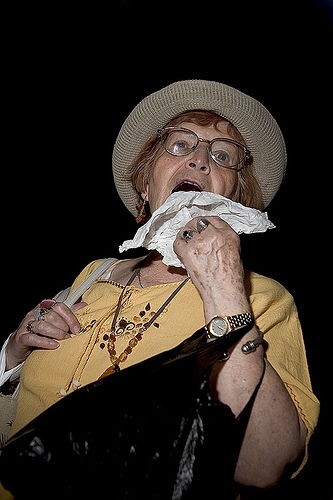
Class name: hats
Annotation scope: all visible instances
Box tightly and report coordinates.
[111,78,288,221]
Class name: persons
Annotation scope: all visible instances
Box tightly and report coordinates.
[0,78,320,500]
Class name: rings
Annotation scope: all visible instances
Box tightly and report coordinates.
[196,218,211,234]
[38,307,52,321]
[182,228,199,242]
[26,320,39,334]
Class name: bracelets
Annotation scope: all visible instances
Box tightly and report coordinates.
[217,338,269,362]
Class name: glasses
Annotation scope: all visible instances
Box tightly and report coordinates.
[153,125,252,169]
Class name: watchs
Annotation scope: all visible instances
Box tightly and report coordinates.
[204,311,255,340]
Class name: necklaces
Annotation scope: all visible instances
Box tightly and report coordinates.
[97,255,192,381]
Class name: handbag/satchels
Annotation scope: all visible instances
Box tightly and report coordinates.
[1,258,122,444]
[0,320,266,500]
